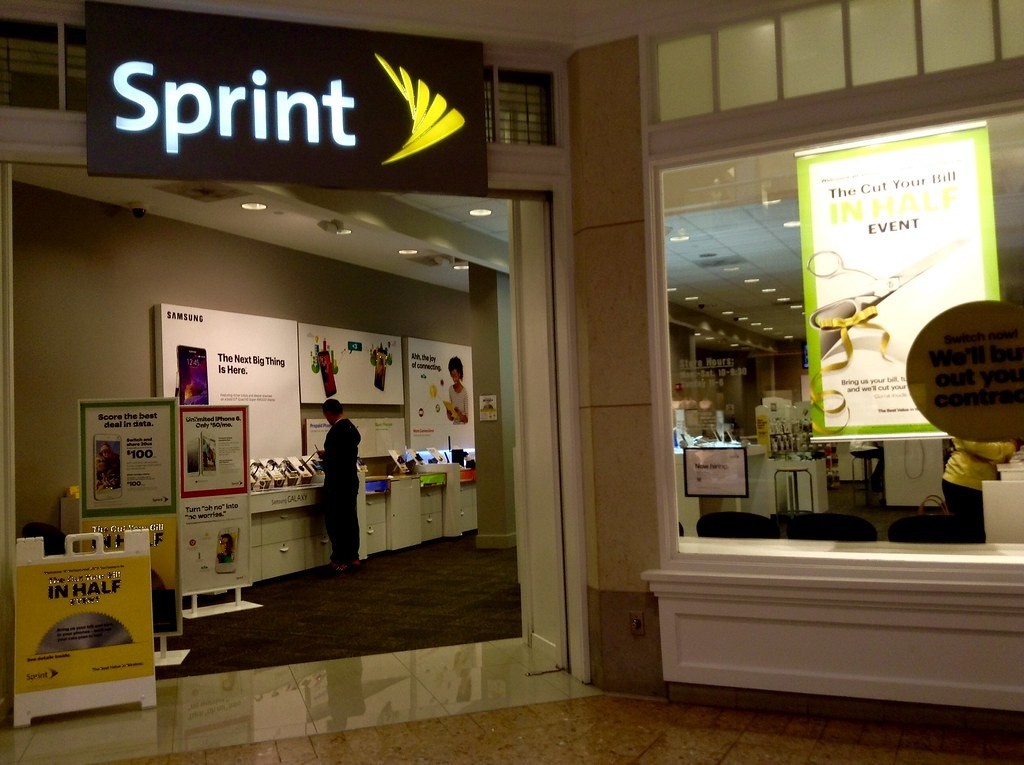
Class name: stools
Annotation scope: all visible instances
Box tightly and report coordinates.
[774,466,814,519]
[851,447,884,508]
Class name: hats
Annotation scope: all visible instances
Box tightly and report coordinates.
[99,445,111,453]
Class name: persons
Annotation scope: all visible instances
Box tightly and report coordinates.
[941,434,1022,530]
[96,442,121,494]
[445,356,469,424]
[216,534,238,566]
[316,398,362,572]
[849,441,886,492]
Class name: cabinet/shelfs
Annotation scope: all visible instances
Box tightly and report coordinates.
[305,503,333,570]
[459,480,478,532]
[262,506,305,581]
[420,485,443,542]
[365,494,387,555]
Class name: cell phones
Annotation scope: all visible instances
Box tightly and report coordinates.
[317,351,337,399]
[177,345,209,406]
[348,341,362,351]
[374,352,386,391]
[93,435,121,500]
[188,431,217,477]
[215,527,239,575]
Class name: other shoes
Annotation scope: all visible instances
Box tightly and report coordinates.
[325,562,339,572]
[333,560,361,577]
